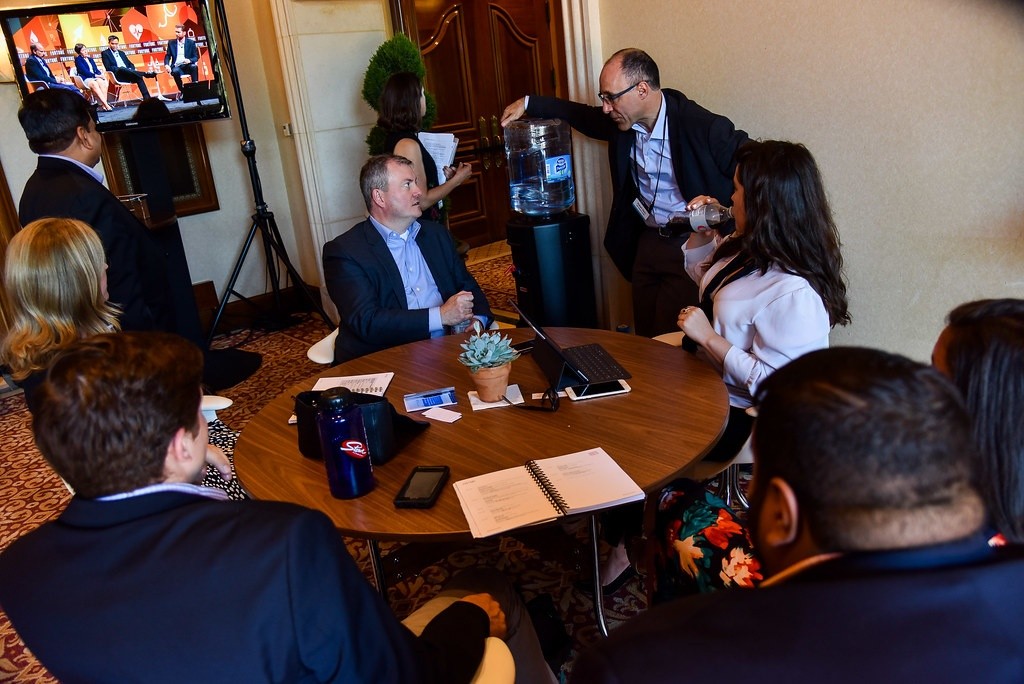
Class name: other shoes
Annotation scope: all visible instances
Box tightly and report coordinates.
[102,106,107,111]
[603,563,637,595]
[108,107,112,111]
[179,91,183,100]
[145,72,157,77]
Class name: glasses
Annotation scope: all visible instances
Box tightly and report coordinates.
[598,80,650,104]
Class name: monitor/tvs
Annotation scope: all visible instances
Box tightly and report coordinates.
[0,0,232,134]
[182,80,218,103]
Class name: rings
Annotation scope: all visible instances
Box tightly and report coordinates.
[683,307,690,314]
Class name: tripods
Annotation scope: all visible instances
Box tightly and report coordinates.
[207,0,336,347]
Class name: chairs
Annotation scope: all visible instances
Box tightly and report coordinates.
[167,62,198,101]
[69,67,115,109]
[106,71,144,108]
[24,74,50,92]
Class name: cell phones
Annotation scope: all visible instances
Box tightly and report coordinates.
[393,466,451,509]
[511,338,535,354]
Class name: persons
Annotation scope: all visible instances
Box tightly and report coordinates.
[16,88,202,352]
[568,346,1024,684]
[74,43,113,112]
[101,36,157,99]
[369,71,474,225]
[164,24,200,100]
[3,216,249,503]
[497,47,754,340]
[25,42,98,109]
[323,155,495,368]
[1,334,557,684]
[598,140,853,591]
[929,298,1024,543]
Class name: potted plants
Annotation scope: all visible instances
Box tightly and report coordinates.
[455,321,522,402]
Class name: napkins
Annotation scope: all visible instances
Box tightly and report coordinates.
[467,384,525,412]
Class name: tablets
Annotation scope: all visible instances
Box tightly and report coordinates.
[506,298,587,385]
[565,379,631,401]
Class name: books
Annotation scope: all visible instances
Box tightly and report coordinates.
[453,446,647,540]
[288,371,395,425]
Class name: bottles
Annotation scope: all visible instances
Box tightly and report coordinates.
[658,204,732,238]
[504,119,575,221]
[453,320,472,335]
[317,387,376,499]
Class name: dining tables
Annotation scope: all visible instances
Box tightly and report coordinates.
[232,327,732,639]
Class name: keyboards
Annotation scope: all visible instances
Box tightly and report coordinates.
[564,343,632,383]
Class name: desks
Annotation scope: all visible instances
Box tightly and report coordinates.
[146,70,172,101]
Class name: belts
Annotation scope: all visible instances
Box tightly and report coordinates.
[651,227,688,238]
